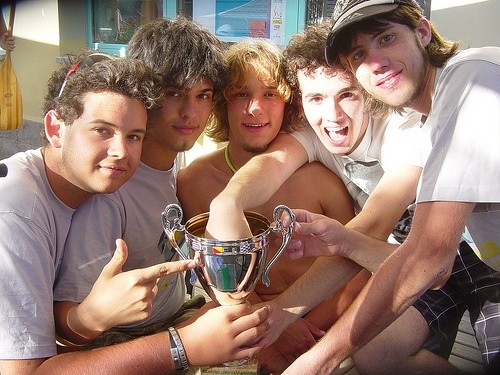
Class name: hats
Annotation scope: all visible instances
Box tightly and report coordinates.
[324,0,420,65]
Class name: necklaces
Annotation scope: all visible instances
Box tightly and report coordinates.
[224,143,238,174]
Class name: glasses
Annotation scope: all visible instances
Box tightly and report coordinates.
[58,53,123,99]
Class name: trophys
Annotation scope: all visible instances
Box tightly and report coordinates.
[161,203,296,375]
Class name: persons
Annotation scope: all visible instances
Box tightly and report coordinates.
[204,18,500,375]
[271,0,500,375]
[53,17,231,375]
[0,48,274,375]
[176,39,372,375]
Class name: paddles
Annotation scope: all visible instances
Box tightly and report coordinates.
[0,0,23,130]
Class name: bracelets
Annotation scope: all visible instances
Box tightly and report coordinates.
[167,326,189,375]
